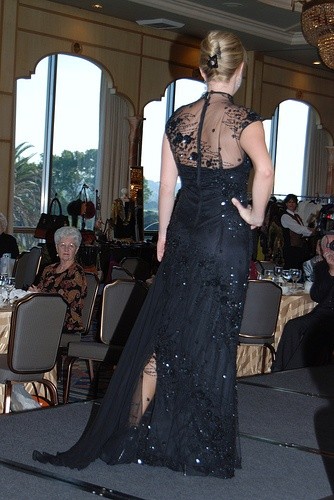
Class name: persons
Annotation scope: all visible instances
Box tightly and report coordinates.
[281,194,314,269]
[146,255,160,285]
[0,212,19,259]
[248,200,270,261]
[149,234,158,247]
[28,226,87,381]
[271,230,334,372]
[261,221,289,258]
[33,30,273,478]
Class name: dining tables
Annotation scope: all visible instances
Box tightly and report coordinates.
[82,240,150,269]
[237,283,319,379]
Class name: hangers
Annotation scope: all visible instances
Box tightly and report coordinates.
[301,196,320,207]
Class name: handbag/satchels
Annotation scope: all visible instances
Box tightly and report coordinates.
[34,198,70,239]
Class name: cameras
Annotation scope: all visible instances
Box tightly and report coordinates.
[329,240,334,250]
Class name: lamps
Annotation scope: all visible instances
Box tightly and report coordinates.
[291,0,334,70]
[136,11,185,29]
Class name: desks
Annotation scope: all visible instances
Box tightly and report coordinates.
[0,291,52,352]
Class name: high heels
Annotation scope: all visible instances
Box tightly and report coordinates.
[116,425,138,462]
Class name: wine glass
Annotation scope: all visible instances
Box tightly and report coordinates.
[264,266,302,290]
[0,273,16,306]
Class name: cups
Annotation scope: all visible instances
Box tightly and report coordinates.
[274,279,287,287]
[281,287,288,294]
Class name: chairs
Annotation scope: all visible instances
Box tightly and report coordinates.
[13,247,42,288]
[63,255,141,403]
[238,280,282,374]
[0,294,69,412]
